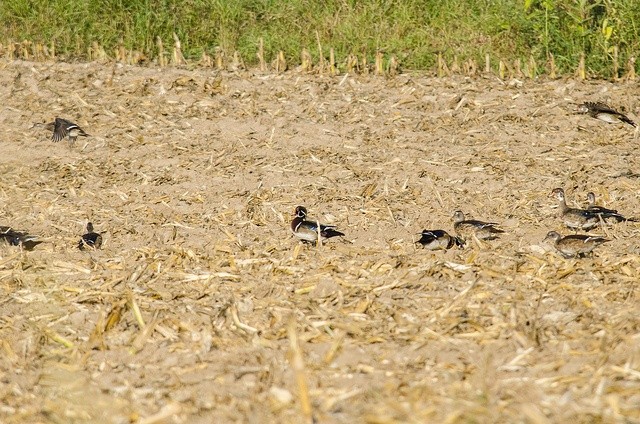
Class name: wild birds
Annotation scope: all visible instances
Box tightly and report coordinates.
[1,221,55,252]
[413,209,505,257]
[45,116,92,147]
[77,221,104,253]
[569,97,640,131]
[289,204,345,248]
[540,183,636,260]
